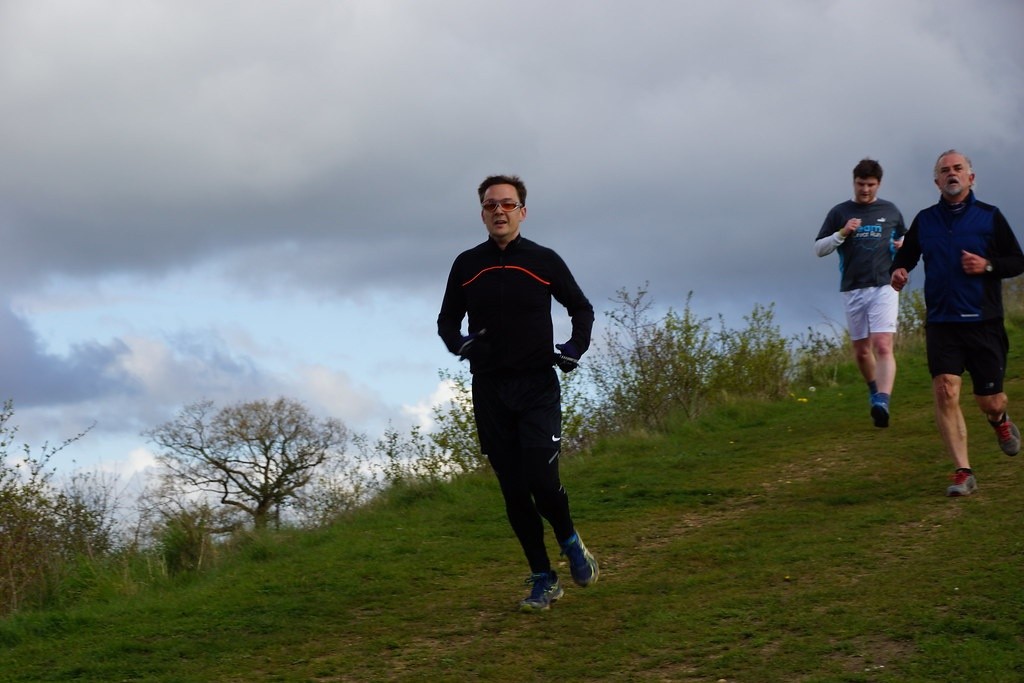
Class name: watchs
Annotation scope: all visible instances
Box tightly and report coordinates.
[985,260,993,272]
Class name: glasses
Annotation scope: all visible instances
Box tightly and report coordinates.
[481,197,523,212]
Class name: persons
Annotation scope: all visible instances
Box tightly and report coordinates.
[889,149,1024,495]
[815,160,907,428]
[438,176,595,611]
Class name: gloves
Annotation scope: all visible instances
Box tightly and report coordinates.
[459,328,486,363]
[556,342,581,373]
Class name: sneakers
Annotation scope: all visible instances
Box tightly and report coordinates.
[870,393,890,428]
[518,570,564,613]
[560,529,600,588]
[986,414,1021,457]
[946,470,977,497]
